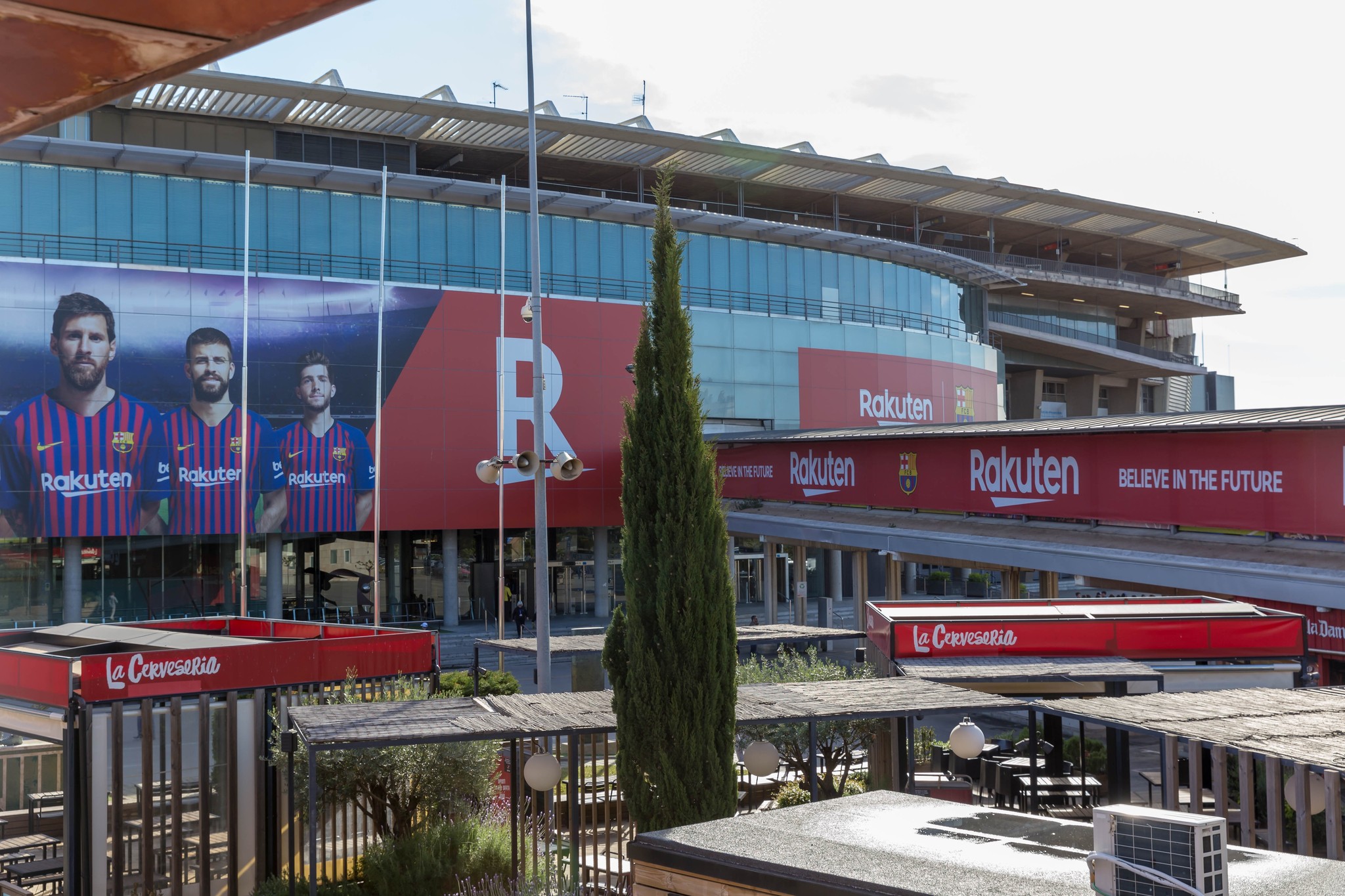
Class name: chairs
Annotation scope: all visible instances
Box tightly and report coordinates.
[579,849,626,896]
[141,784,173,818]
[540,838,570,889]
[927,742,1075,812]
[180,780,214,812]
[36,793,64,834]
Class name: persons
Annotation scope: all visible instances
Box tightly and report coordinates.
[407,594,436,619]
[144,328,287,534]
[1075,591,1155,598]
[0,293,171,537]
[577,566,586,582]
[531,612,536,624]
[748,615,759,659]
[512,601,529,639]
[263,351,375,532]
[749,567,756,598]
[504,581,512,622]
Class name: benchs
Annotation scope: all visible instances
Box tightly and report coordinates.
[0,852,36,873]
[10,872,64,894]
[1018,790,1090,816]
[154,842,198,876]
[753,799,781,814]
[189,858,227,883]
[167,845,228,885]
[1047,802,1104,823]
[0,872,51,891]
[123,826,195,874]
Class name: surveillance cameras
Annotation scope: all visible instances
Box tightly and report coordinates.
[521,305,533,323]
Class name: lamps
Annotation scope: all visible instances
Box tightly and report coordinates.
[949,712,985,758]
[1284,771,1326,815]
[742,722,780,776]
[523,738,562,791]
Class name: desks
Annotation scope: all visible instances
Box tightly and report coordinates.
[0,773,228,896]
[950,743,1000,796]
[1253,828,1292,852]
[562,775,629,828]
[737,756,810,811]
[530,838,571,889]
[1178,787,1216,812]
[816,749,869,775]
[737,791,746,801]
[1139,771,1161,808]
[998,757,1047,812]
[1018,775,1103,815]
[1187,807,1260,847]
[562,853,632,896]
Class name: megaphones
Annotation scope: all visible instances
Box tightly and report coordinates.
[550,452,584,481]
[475,455,502,484]
[512,450,540,477]
